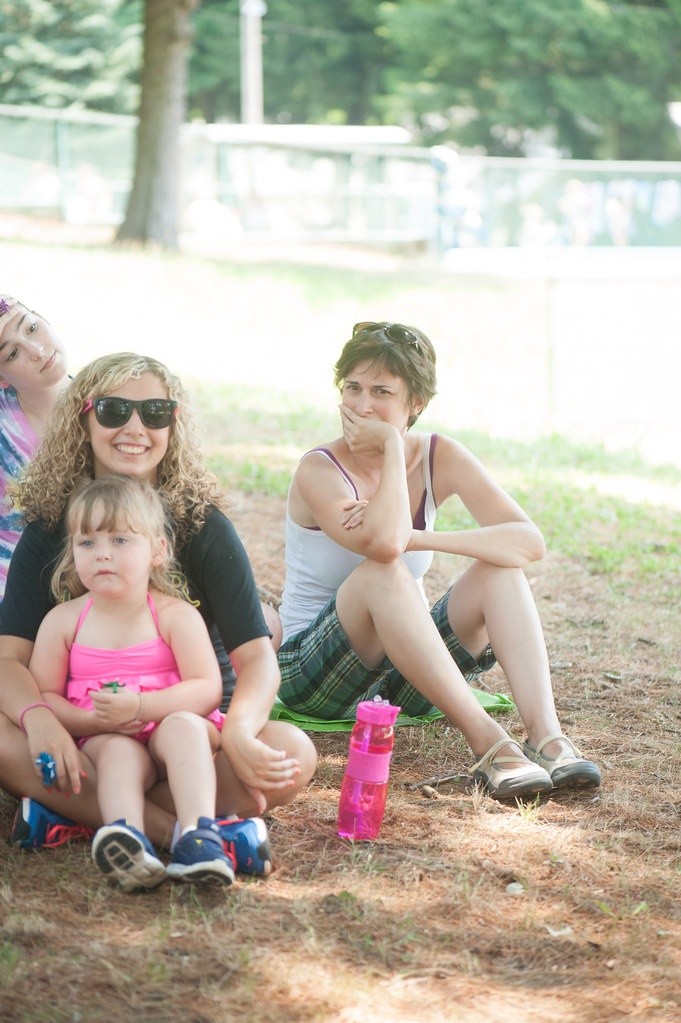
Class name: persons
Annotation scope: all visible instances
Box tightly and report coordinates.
[277,322,601,799]
[0,291,318,892]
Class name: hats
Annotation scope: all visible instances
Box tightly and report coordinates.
[0,296,21,337]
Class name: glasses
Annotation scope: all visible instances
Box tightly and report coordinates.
[81,396,180,429]
[353,321,419,352]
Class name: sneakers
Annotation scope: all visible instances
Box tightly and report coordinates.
[91,818,169,895]
[11,796,92,848]
[166,815,236,885]
[214,814,272,875]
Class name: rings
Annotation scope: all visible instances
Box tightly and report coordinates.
[103,682,127,694]
[37,751,56,786]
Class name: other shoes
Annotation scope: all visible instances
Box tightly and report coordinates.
[468,738,553,799]
[523,733,600,791]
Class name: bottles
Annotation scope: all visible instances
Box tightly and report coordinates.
[337,695,401,841]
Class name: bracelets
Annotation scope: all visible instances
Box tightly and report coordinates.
[18,703,58,734]
[134,693,145,720]
